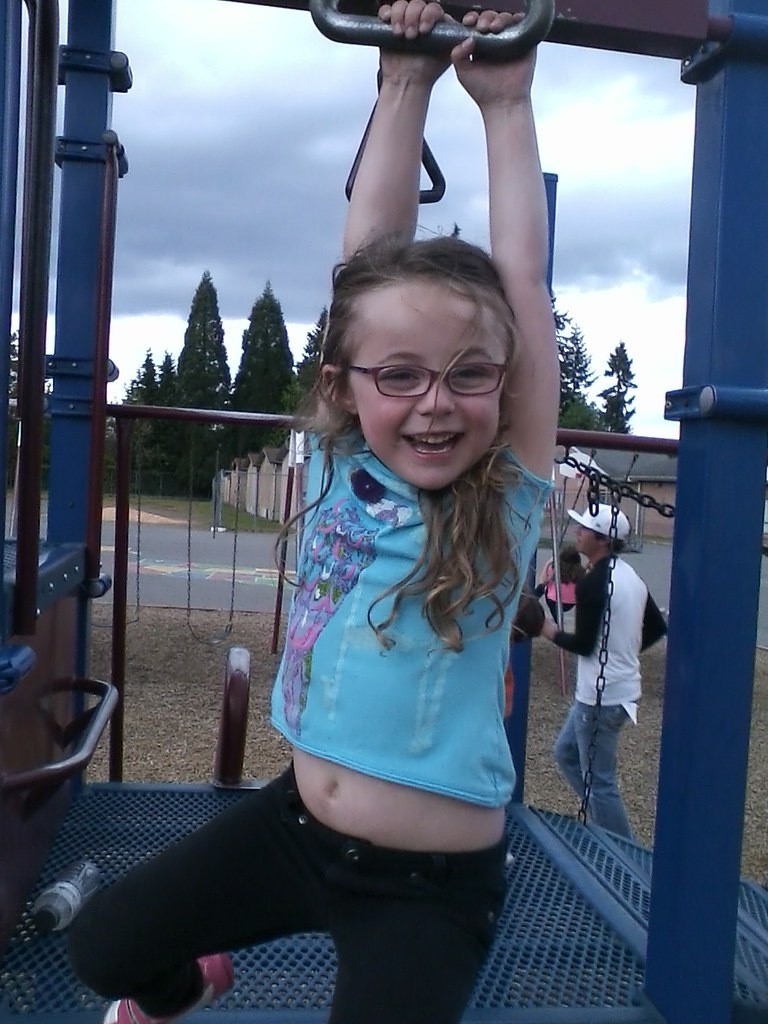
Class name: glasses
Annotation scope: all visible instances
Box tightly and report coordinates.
[347,363,509,396]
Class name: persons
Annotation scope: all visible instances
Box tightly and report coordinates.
[69,0,561,1024]
[544,504,666,838]
[542,545,585,630]
[505,584,546,718]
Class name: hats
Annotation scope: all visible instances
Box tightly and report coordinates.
[567,503,630,541]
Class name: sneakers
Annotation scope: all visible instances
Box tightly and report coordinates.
[103,951,235,1024]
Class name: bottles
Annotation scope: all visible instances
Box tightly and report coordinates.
[34,859,101,932]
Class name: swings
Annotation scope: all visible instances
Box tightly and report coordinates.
[184,420,242,646]
[86,416,146,631]
